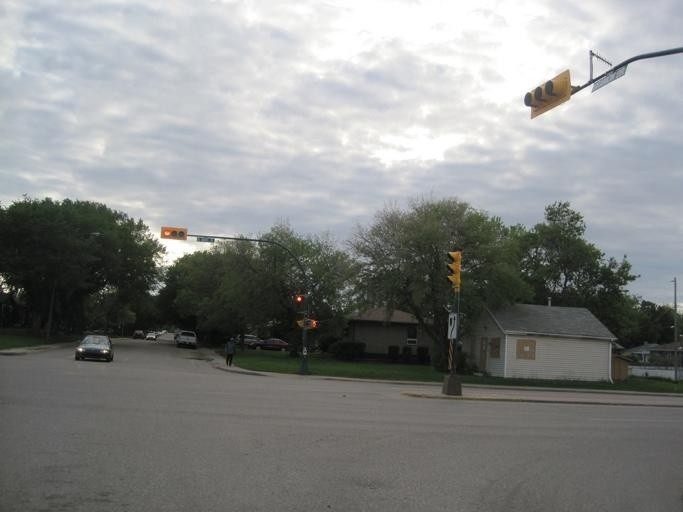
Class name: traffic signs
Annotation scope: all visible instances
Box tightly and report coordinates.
[591,64,628,93]
[197,236,214,243]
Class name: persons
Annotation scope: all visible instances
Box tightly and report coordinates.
[224,337,235,366]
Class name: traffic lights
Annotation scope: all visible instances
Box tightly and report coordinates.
[309,320,319,328]
[524,70,573,119]
[445,251,461,289]
[294,294,306,305]
[161,227,187,240]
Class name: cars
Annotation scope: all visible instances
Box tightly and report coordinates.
[75,335,114,362]
[231,333,292,352]
[133,330,197,349]
